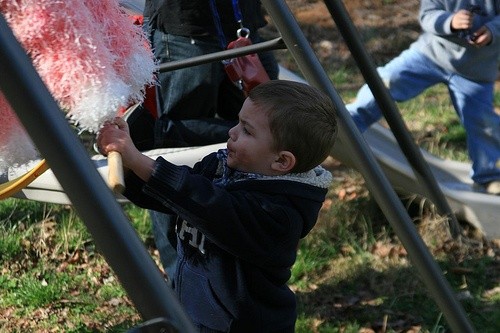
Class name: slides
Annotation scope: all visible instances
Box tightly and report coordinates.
[274,62,500,239]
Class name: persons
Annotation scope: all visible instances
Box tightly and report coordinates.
[141,0,280,294]
[97,79,339,333]
[340,0,500,197]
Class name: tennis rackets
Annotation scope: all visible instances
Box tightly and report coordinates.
[99,20,151,194]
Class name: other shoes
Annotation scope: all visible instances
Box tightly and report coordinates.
[475,177,500,196]
